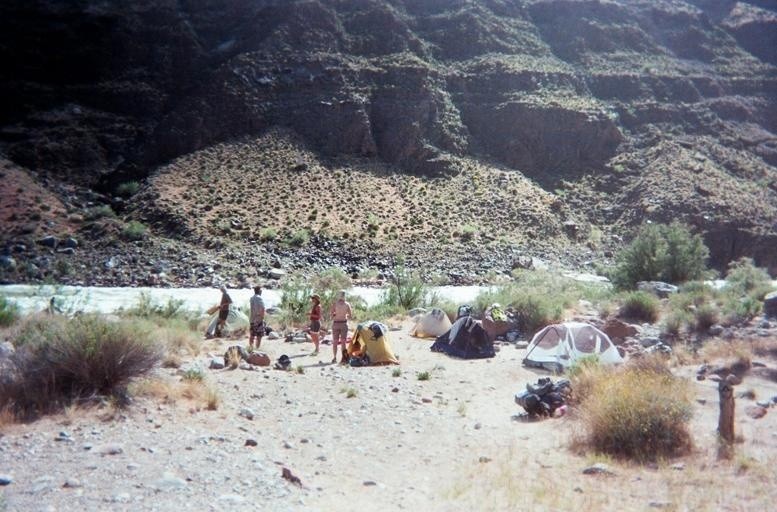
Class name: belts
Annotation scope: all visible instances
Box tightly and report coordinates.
[335,321,346,323]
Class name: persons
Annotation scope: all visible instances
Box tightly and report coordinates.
[329,289,351,364]
[303,293,321,356]
[246,285,266,348]
[213,283,234,337]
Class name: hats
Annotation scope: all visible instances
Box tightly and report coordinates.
[311,295,320,303]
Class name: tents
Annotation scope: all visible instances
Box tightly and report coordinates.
[520,322,625,377]
[342,321,400,368]
[407,306,452,340]
[429,315,496,360]
